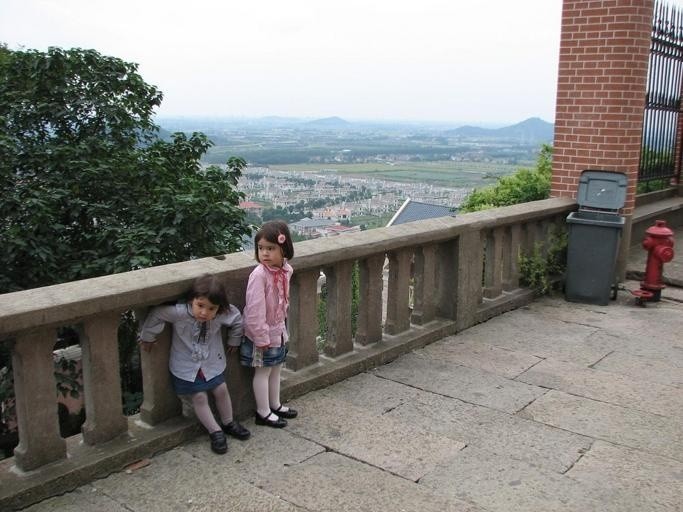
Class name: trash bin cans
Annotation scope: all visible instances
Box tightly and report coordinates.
[564,170,627,305]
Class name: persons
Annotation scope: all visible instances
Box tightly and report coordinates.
[236,220,298,428]
[139,275,250,455]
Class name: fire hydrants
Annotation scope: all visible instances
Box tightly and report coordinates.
[630,221,674,305]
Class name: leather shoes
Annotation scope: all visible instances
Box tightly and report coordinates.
[209,422,250,454]
[255,404,297,428]
